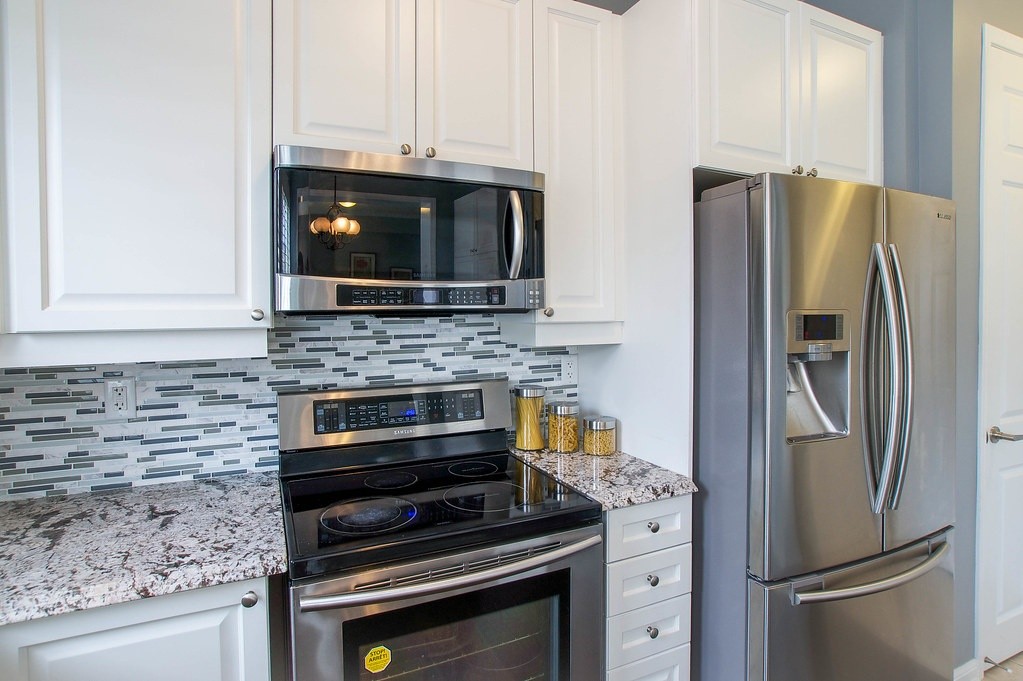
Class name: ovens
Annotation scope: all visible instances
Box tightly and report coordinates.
[288,519,606,680]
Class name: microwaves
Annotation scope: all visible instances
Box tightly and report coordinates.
[273,145,547,312]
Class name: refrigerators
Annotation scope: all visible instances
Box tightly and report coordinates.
[692,173,954,681]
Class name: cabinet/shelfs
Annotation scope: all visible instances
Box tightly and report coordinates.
[0,579,271,679]
[606,492,690,681]
[502,0,623,347]
[3,1,270,373]
[272,1,533,170]
[621,1,885,477]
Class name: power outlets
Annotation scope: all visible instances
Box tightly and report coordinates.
[560,355,578,385]
[104,376,136,419]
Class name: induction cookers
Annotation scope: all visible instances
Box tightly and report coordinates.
[276,380,603,579]
